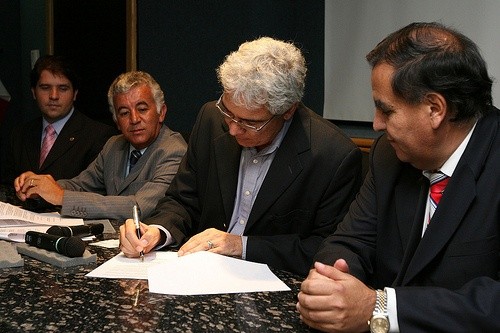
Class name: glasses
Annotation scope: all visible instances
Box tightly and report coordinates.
[216,93,275,132]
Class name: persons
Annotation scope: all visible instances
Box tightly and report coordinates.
[297,22,500,333]
[14,72,189,220]
[119,37,363,277]
[0,56,121,189]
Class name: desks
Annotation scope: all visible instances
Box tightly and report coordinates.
[1,190,329,333]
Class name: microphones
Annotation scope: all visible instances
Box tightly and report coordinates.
[46,222,104,238]
[25,230,86,259]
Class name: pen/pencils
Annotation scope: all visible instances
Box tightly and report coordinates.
[133,201,144,261]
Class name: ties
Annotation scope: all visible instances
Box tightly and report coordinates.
[427,173,451,228]
[39,124,56,169]
[129,150,143,176]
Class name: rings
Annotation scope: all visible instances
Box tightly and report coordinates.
[208,241,213,248]
[29,179,34,186]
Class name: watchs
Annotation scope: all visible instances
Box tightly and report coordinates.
[368,289,390,333]
